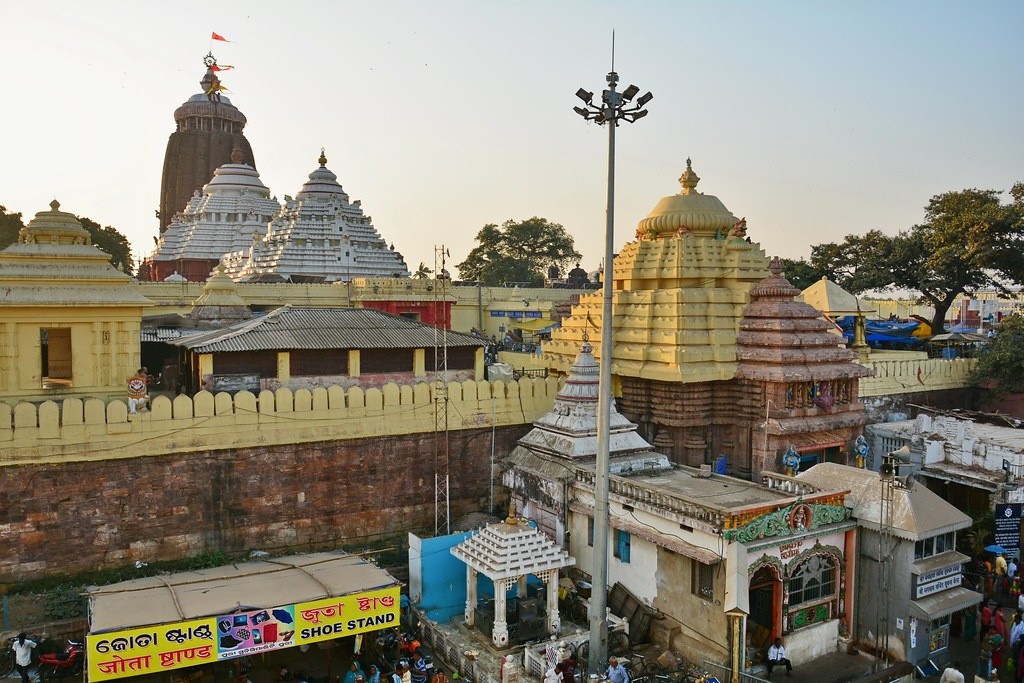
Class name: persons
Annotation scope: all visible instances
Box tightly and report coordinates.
[239,671,252,683]
[344,651,449,683]
[767,637,792,681]
[141,367,150,411]
[888,313,900,322]
[12,633,38,683]
[484,335,550,365]
[988,309,1013,323]
[940,552,1024,683]
[987,328,994,338]
[542,654,578,683]
[746,236,751,244]
[605,656,629,683]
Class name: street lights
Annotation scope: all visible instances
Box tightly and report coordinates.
[573,28,654,675]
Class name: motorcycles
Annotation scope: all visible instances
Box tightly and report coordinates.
[34,639,84,683]
[376,628,426,657]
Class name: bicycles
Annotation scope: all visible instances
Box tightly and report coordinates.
[620,654,700,683]
[0,637,46,680]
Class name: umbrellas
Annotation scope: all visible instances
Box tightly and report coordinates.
[984,544,1007,552]
